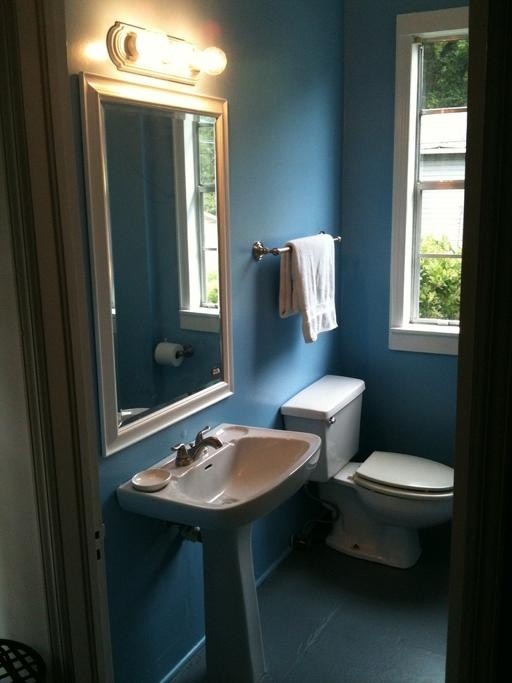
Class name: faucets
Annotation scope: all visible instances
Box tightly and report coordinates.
[190,436,222,460]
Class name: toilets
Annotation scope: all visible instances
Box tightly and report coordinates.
[282,372,456,569]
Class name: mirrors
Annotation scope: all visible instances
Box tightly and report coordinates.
[80,71,234,456]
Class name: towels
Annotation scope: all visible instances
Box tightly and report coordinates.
[278,233,339,343]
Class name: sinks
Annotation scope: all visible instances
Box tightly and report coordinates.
[116,423,322,528]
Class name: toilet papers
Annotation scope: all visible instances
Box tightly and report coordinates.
[155,342,183,368]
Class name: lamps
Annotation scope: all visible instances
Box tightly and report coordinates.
[105,21,228,86]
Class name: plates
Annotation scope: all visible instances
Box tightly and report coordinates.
[132,468,171,490]
